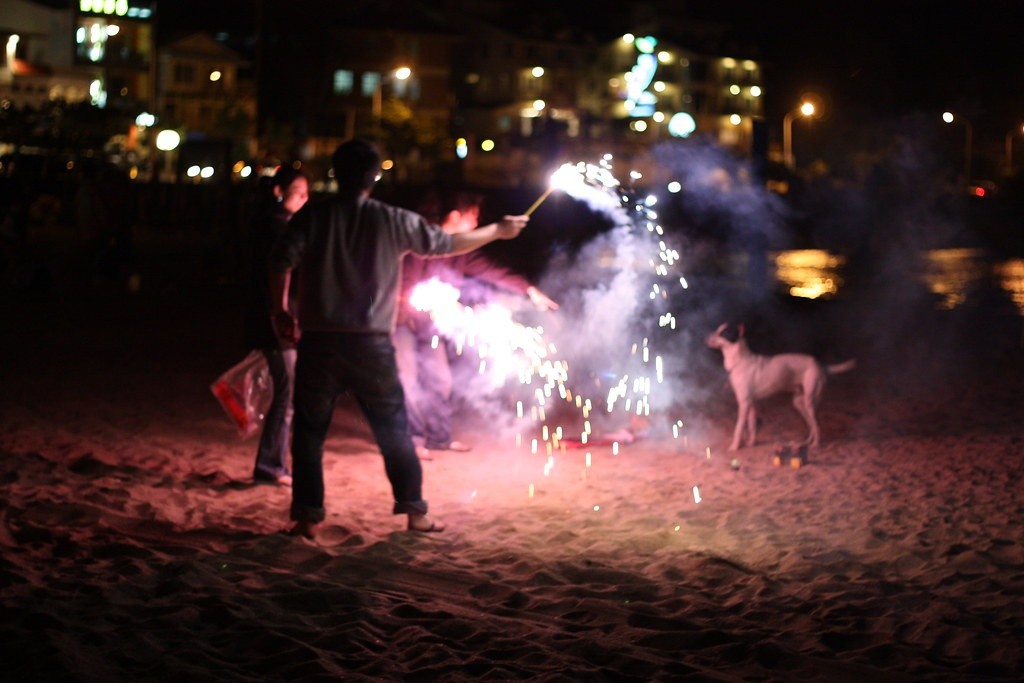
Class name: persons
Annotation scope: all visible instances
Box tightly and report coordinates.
[270,138,531,541]
[240,164,309,488]
[391,173,562,453]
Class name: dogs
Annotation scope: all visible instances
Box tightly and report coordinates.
[707,319,855,452]
